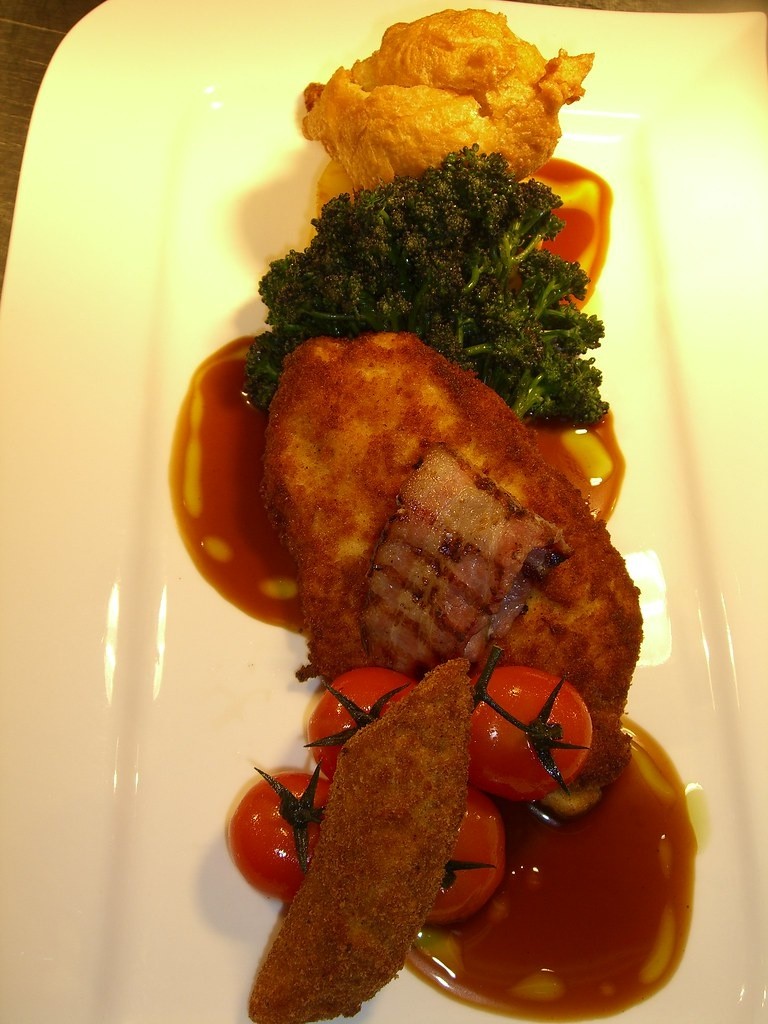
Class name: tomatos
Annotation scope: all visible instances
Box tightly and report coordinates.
[229,667,592,924]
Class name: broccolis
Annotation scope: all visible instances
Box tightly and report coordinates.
[241,144,611,428]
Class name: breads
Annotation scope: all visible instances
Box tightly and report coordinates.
[302,7,595,193]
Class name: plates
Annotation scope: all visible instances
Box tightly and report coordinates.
[0,1,768,1024]
